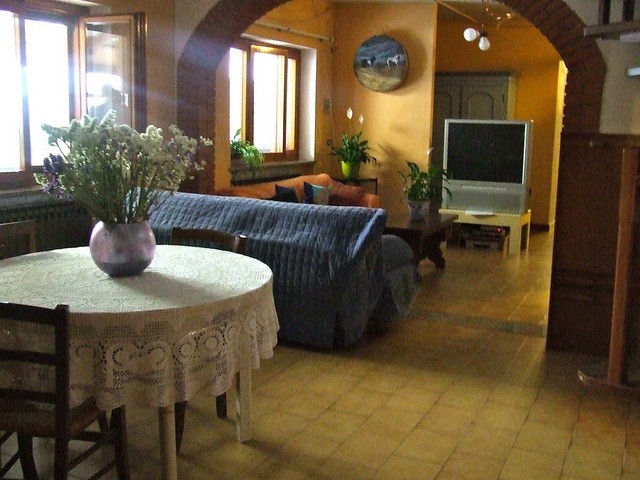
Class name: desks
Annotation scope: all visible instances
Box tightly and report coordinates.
[1,239,281,480]
[438,208,531,257]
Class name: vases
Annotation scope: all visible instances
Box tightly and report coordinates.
[89,218,157,277]
[339,161,361,180]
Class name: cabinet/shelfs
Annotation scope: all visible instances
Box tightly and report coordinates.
[432,74,518,197]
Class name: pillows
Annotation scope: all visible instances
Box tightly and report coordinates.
[274,184,300,203]
[302,180,332,205]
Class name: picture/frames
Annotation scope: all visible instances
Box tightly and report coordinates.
[352,34,409,93]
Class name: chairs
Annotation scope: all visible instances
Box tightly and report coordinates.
[1,300,131,480]
[159,227,246,456]
[0,220,36,261]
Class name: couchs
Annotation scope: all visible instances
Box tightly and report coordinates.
[215,173,382,209]
[119,186,421,353]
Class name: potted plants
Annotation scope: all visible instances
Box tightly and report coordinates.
[229,128,264,169]
[396,160,453,222]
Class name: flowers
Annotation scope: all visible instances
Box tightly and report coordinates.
[33,109,213,226]
[330,106,377,167]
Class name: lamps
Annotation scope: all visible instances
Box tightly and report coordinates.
[463,23,490,51]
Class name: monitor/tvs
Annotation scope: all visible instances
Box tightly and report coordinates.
[445,119,531,185]
